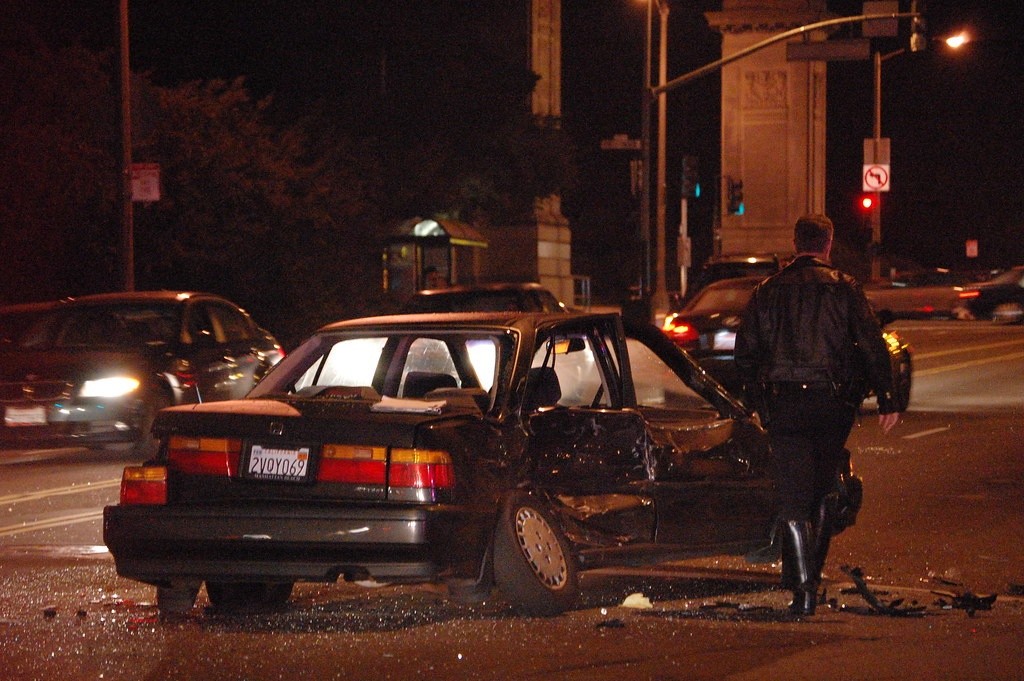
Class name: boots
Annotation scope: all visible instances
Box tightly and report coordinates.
[783,514,818,616]
[787,504,831,608]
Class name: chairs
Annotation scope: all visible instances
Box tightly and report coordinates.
[403,370,456,402]
[85,312,132,347]
[521,367,561,414]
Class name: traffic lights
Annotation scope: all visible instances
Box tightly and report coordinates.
[725,176,745,214]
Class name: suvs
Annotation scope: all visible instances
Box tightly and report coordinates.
[397,284,579,317]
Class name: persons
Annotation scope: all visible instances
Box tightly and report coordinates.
[734,214,893,617]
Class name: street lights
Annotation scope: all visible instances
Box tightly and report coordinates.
[646,1,927,329]
[867,32,965,286]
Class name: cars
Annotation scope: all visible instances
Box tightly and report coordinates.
[102,306,863,614]
[948,265,1023,327]
[0,286,288,460]
[659,275,915,419]
